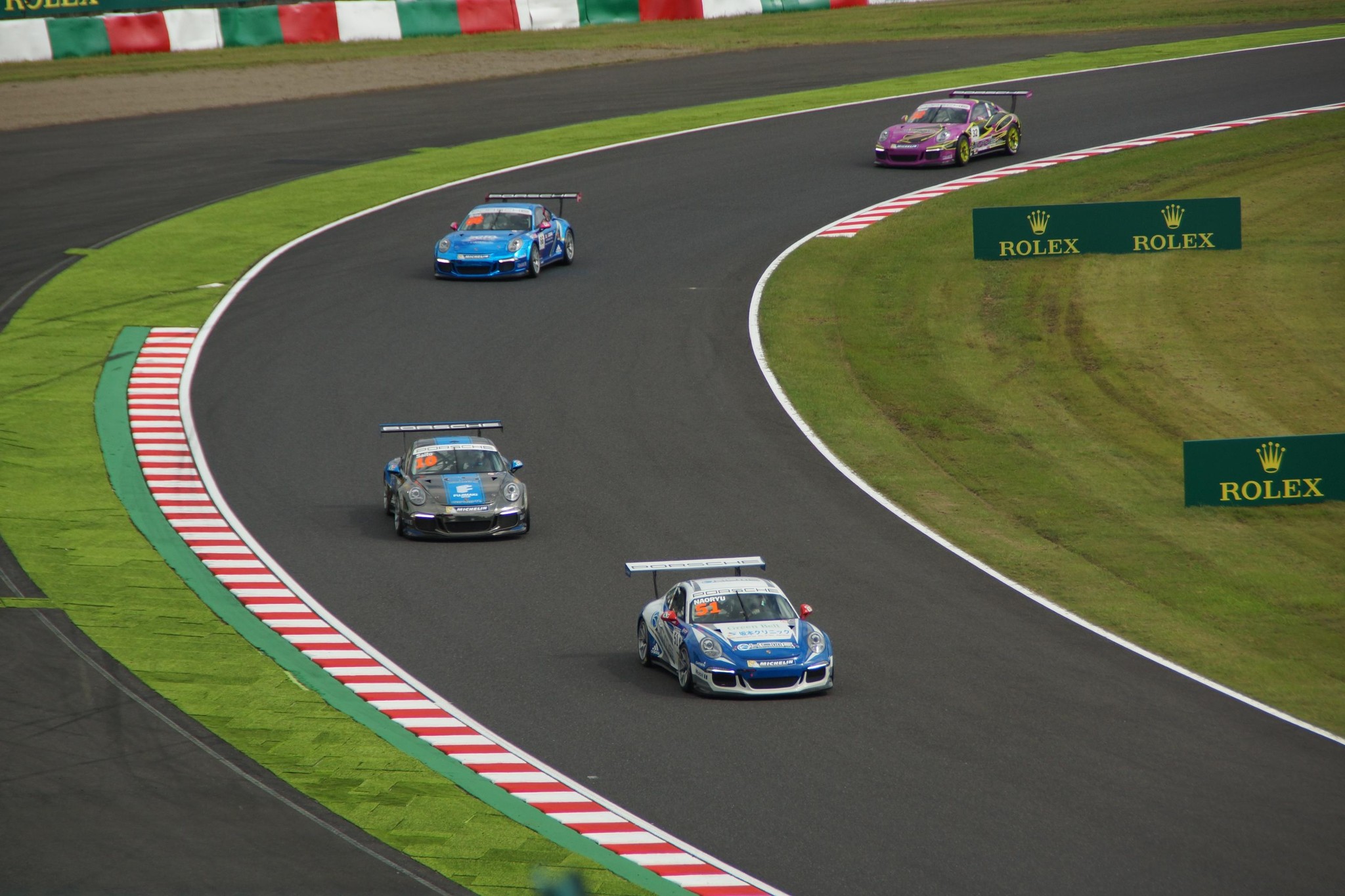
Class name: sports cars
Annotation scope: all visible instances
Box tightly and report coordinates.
[375,419,530,540]
[623,555,834,701]
[873,88,1030,169]
[432,189,583,283]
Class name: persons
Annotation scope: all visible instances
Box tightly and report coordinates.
[463,450,492,472]
[745,594,773,621]
